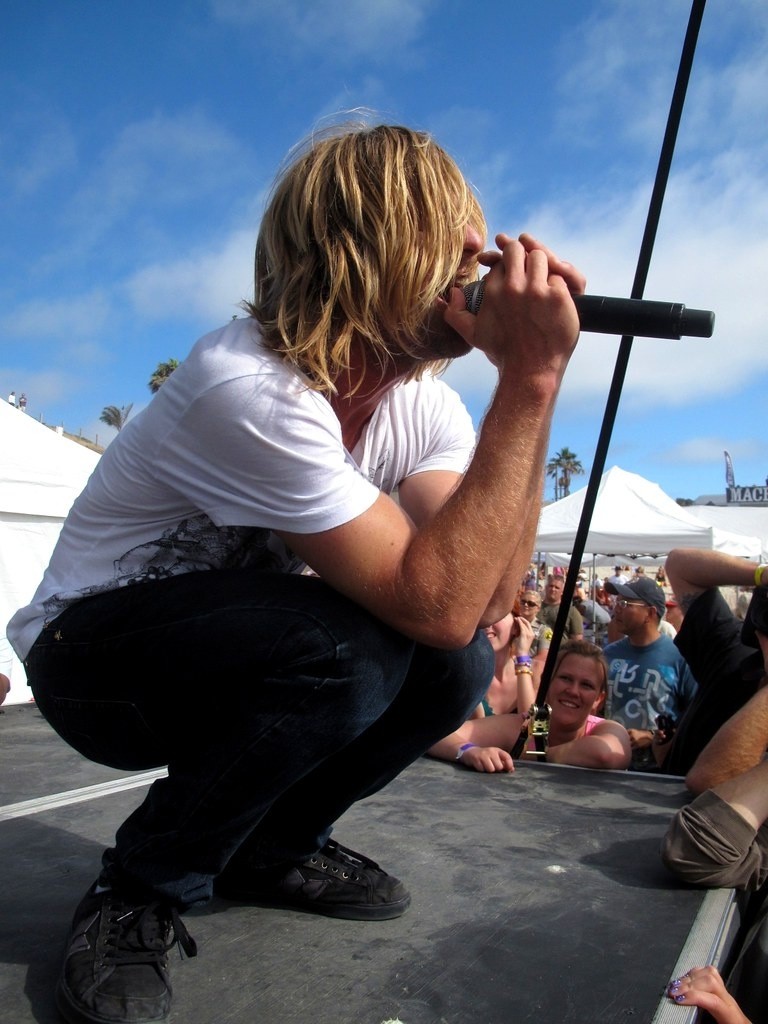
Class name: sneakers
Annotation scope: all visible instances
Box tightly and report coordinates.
[55,873,201,1024]
[224,834,411,920]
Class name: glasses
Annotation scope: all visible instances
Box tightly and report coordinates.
[616,599,645,609]
[519,599,539,608]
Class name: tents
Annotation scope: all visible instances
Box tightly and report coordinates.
[533,465,762,648]
[0,397,102,707]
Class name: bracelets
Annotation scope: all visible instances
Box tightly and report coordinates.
[755,564,768,584]
[455,744,476,766]
[513,655,533,675]
[649,730,654,735]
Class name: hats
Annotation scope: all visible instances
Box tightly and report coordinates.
[604,576,667,619]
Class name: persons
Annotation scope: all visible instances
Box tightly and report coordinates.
[9,392,15,406]
[5,125,586,1024]
[465,561,768,775]
[658,547,768,1023]
[19,393,27,412]
[423,641,632,773]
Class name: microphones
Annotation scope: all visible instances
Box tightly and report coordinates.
[462,280,715,341]
